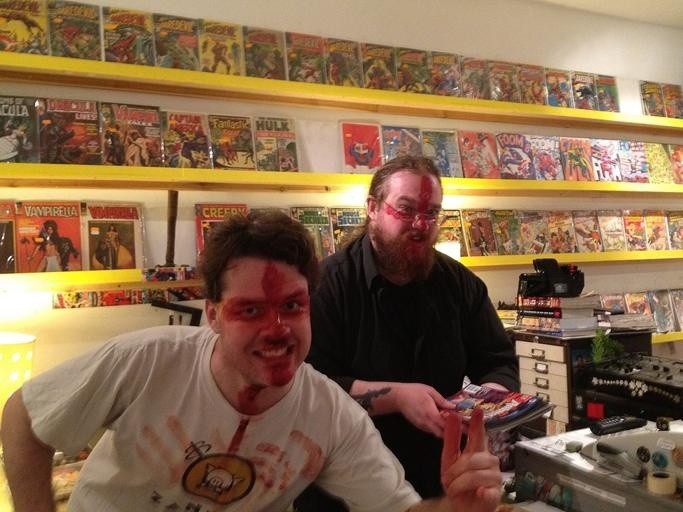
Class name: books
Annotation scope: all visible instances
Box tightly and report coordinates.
[437,382,556,438]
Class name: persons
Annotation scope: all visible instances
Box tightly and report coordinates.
[293,154,520,512]
[1,210,505,512]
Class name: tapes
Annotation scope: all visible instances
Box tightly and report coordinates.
[646,470,679,495]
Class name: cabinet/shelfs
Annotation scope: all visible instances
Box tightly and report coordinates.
[515,337,653,423]
[2,50,681,347]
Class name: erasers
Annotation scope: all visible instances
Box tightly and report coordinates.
[566,440,583,452]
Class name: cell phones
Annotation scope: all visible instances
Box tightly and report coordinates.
[588,414,648,436]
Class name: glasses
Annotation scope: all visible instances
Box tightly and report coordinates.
[379,196,448,227]
